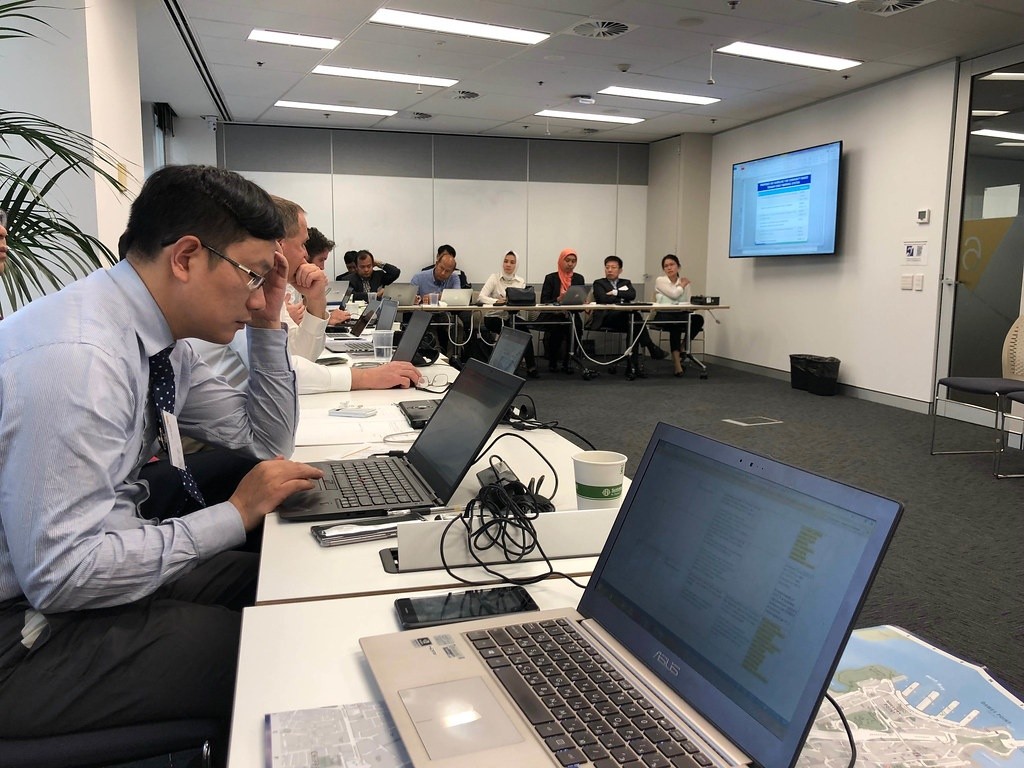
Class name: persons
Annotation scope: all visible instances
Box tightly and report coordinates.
[536,248,587,373]
[0,209,8,320]
[650,254,704,375]
[0,164,299,768]
[410,244,476,362]
[303,227,351,325]
[477,250,539,378]
[183,195,425,390]
[590,256,669,382]
[335,251,401,301]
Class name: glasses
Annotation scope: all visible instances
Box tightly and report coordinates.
[160,235,267,290]
[415,374,449,389]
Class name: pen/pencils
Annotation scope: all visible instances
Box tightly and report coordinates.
[334,338,362,340]
[500,294,504,298]
[351,327,375,328]
[387,504,482,516]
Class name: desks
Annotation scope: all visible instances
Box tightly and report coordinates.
[397,303,731,379]
[227,329,633,768]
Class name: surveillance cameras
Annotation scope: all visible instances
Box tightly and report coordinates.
[205,115,219,132]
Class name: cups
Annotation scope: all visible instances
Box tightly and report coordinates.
[428,293,439,306]
[571,450,627,510]
[371,329,394,362]
[367,293,377,304]
[345,302,358,314]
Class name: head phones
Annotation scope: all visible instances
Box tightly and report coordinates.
[411,341,439,367]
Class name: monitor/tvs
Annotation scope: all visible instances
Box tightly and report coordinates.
[728,140,843,258]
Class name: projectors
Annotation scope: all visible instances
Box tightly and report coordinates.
[569,97,595,106]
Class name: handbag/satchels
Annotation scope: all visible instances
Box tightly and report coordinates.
[505,285,536,306]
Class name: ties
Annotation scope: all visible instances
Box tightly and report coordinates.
[142,344,207,510]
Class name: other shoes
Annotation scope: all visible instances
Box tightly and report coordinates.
[528,370,540,379]
[562,365,571,374]
[672,365,684,377]
[625,366,650,378]
[650,346,669,361]
[548,363,560,373]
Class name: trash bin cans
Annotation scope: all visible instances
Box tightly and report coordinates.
[806,357,841,397]
[790,354,822,391]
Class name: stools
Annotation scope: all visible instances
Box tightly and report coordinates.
[0,712,231,768]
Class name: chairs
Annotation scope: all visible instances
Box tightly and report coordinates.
[931,313,1024,479]
[402,283,705,367]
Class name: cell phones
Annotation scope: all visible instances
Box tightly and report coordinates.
[394,585,541,630]
[311,513,429,546]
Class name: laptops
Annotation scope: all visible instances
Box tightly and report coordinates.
[359,421,906,768]
[277,357,527,521]
[398,327,533,430]
[558,283,595,305]
[325,282,435,365]
[441,289,474,306]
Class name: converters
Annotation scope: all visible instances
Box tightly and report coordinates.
[476,460,524,508]
